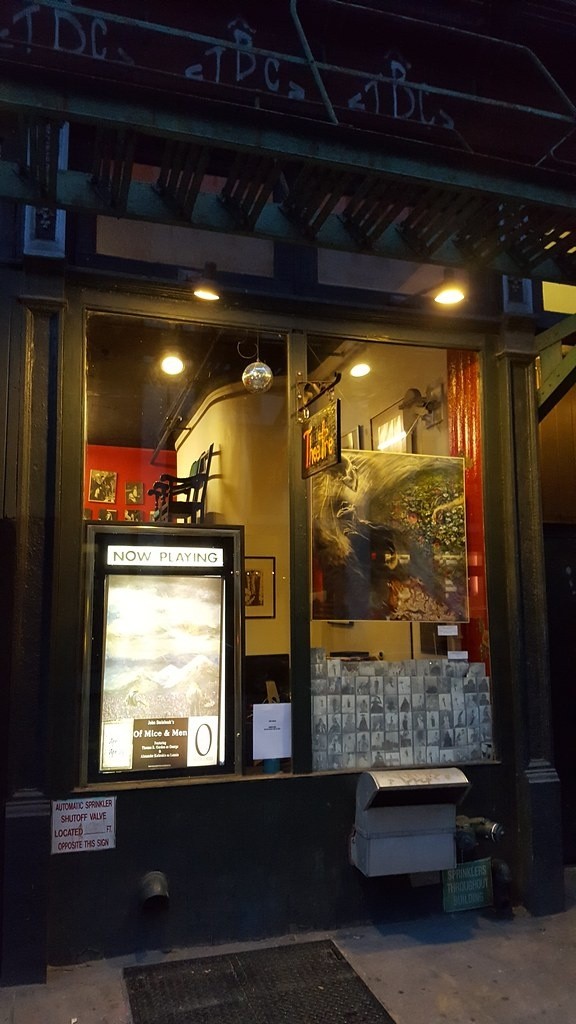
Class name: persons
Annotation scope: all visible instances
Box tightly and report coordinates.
[83,475,142,522]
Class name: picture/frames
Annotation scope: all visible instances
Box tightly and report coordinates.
[88,469,117,503]
[340,425,360,450]
[409,621,456,659]
[245,556,276,619]
[369,395,414,454]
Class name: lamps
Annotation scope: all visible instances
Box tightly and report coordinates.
[402,276,466,304]
[399,388,439,409]
[174,260,221,300]
[238,336,273,395]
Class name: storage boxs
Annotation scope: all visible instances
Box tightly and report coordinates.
[440,856,494,913]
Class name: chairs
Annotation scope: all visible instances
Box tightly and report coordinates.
[147,443,214,524]
